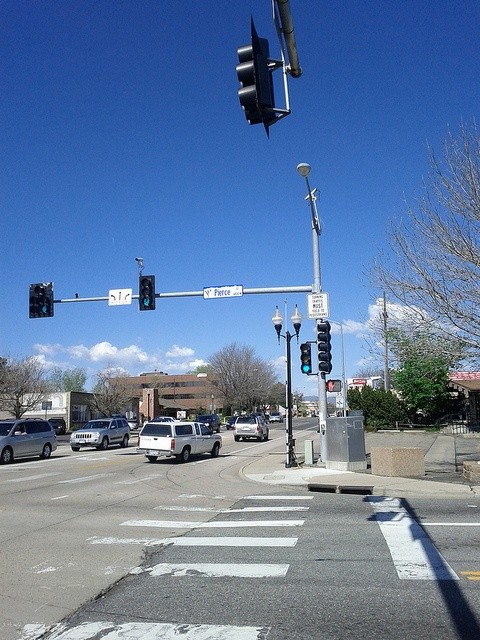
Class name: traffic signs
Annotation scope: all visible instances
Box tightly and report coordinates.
[203,285,243,300]
[108,288,132,306]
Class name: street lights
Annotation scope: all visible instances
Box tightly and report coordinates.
[272,298,302,468]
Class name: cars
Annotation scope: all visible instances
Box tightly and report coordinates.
[128,415,184,430]
[226,416,239,430]
[0,418,57,464]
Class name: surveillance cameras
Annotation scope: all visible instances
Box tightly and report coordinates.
[135,257,144,275]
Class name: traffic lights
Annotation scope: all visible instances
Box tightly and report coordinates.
[234,15,277,137]
[327,382,341,393]
[139,275,155,311]
[29,282,55,318]
[300,343,312,375]
[317,322,331,379]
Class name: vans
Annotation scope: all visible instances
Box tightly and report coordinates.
[137,422,222,463]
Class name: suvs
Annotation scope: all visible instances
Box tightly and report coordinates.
[233,416,269,441]
[193,414,221,433]
[70,417,131,451]
[269,412,283,423]
[49,416,66,434]
